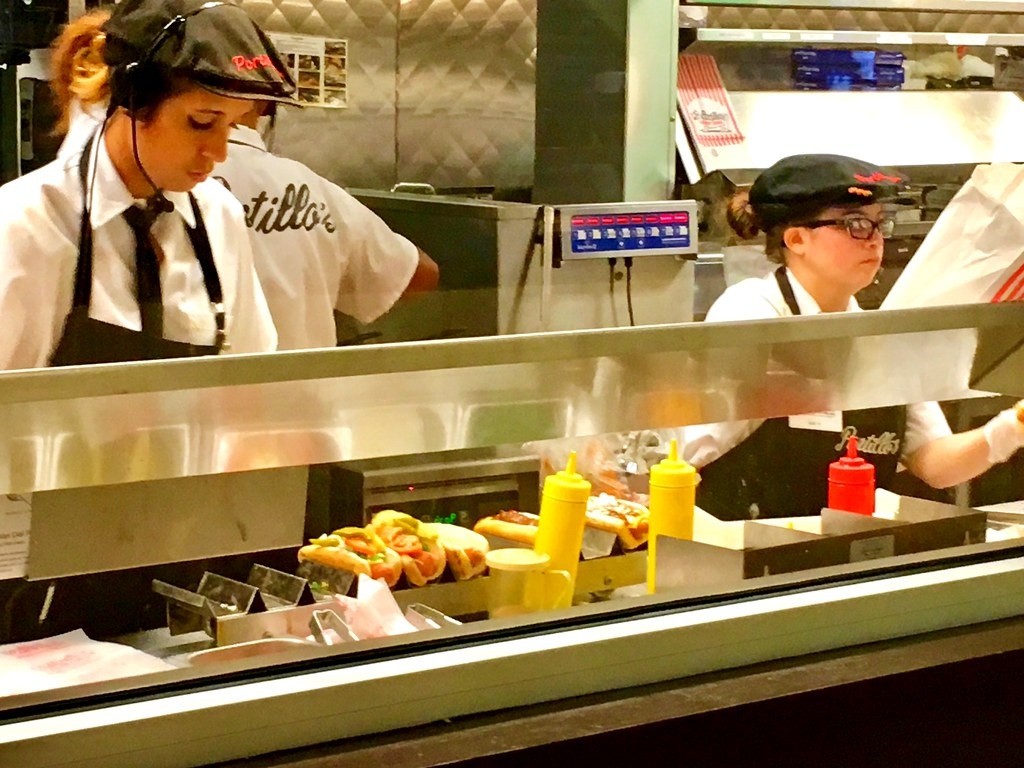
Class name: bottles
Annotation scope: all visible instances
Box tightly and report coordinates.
[533,450,592,607]
[827,434,878,516]
[646,440,703,595]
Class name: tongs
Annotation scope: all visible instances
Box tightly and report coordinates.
[308,609,359,645]
[405,603,462,630]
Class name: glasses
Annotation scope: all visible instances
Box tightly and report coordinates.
[796,209,896,240]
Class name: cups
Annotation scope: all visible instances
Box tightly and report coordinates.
[485,547,572,620]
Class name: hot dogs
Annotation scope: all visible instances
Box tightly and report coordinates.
[297,509,489,587]
[582,492,651,551]
[473,508,539,546]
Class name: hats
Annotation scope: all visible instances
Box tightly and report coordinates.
[748,154,911,230]
[100,0,304,109]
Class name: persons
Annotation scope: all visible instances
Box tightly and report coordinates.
[683,155,1024,522]
[0,0,284,645]
[45,4,441,349]
